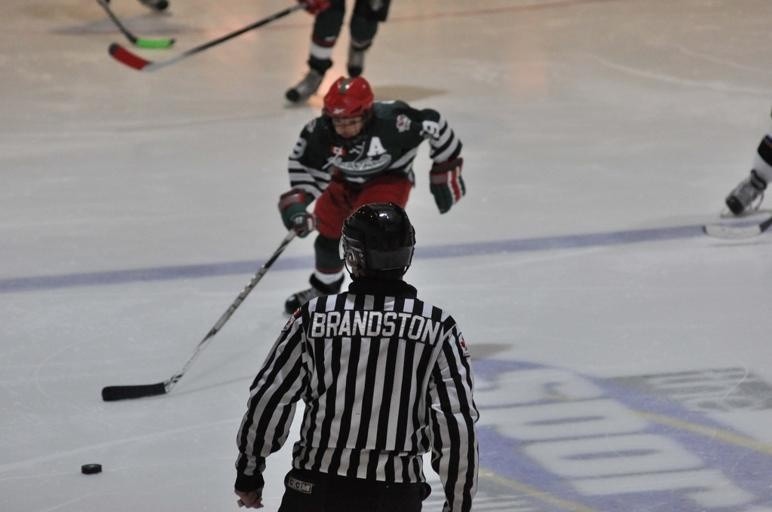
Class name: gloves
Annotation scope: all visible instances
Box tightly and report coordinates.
[279,189,317,237]
[430,158,465,213]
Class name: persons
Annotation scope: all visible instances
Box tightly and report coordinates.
[285,0,391,105]
[277,76,467,315]
[724,132,772,217]
[232,201,482,511]
[96,0,169,11]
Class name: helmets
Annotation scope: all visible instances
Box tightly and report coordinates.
[341,205,414,277]
[325,76,374,145]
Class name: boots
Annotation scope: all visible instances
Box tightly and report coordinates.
[140,0,169,11]
[726,170,767,215]
[285,273,343,315]
[287,58,332,101]
[348,42,370,75]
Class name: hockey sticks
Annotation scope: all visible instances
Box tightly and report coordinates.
[108,2,307,73]
[703,216,772,238]
[97,0,176,49]
[101,227,294,402]
